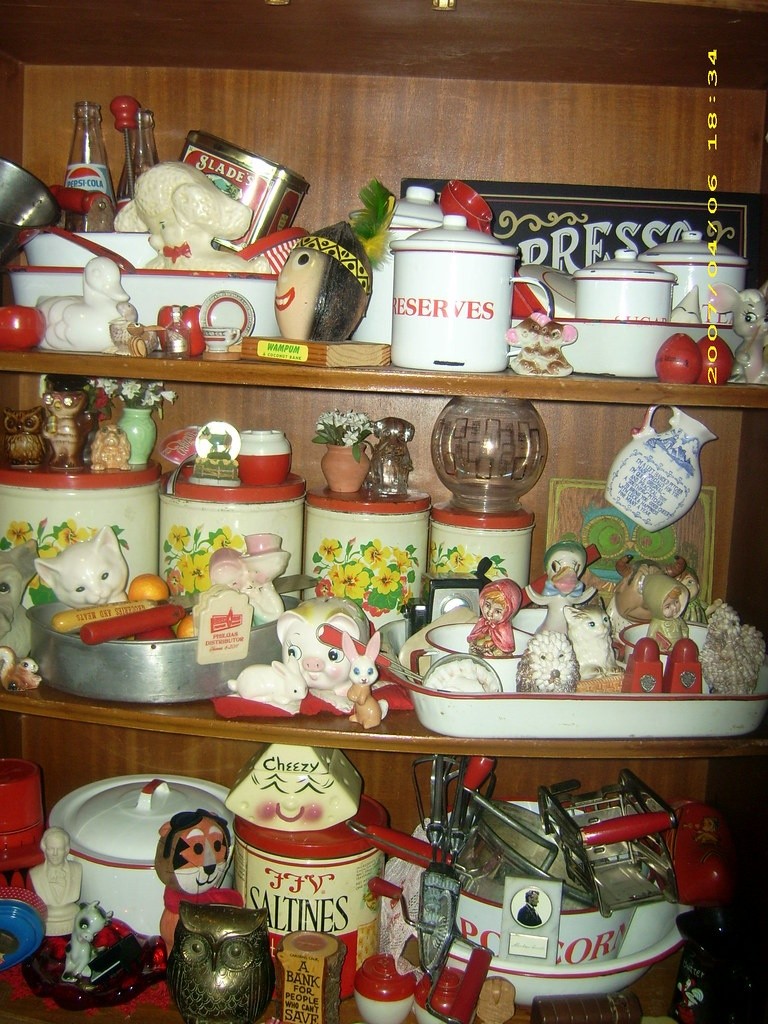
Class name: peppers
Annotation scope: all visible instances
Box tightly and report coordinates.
[158,304,205,355]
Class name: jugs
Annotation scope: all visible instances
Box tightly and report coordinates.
[603,404,719,534]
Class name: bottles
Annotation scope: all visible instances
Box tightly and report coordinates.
[64,101,117,233]
[116,107,159,212]
[164,306,191,361]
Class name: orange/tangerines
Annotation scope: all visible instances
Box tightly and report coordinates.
[177,615,194,637]
[128,574,169,601]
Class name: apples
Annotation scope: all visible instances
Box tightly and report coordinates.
[134,625,175,641]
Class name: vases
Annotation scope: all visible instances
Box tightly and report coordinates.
[323,443,371,492]
[120,408,157,464]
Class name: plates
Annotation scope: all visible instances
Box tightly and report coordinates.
[199,290,255,346]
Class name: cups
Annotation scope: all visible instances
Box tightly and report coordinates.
[571,249,680,322]
[351,187,445,345]
[389,215,519,373]
[637,230,749,323]
[201,326,240,353]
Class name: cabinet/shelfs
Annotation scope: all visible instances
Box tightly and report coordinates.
[0,0,768,1024]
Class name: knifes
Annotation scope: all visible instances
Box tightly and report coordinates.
[53,573,318,646]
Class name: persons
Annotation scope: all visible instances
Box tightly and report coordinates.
[466,579,523,657]
[517,890,542,927]
[28,826,83,936]
[642,572,690,653]
[674,566,709,624]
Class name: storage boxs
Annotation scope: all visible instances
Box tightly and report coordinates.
[179,129,310,254]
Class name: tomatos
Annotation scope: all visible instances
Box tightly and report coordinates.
[0,305,45,351]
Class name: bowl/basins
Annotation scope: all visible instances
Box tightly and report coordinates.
[439,180,493,231]
[443,905,697,1007]
[22,231,160,268]
[458,800,638,966]
[511,607,549,635]
[619,620,715,694]
[48,773,237,935]
[0,157,61,267]
[424,623,536,691]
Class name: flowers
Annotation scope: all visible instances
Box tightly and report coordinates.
[312,409,380,462]
[87,378,177,420]
